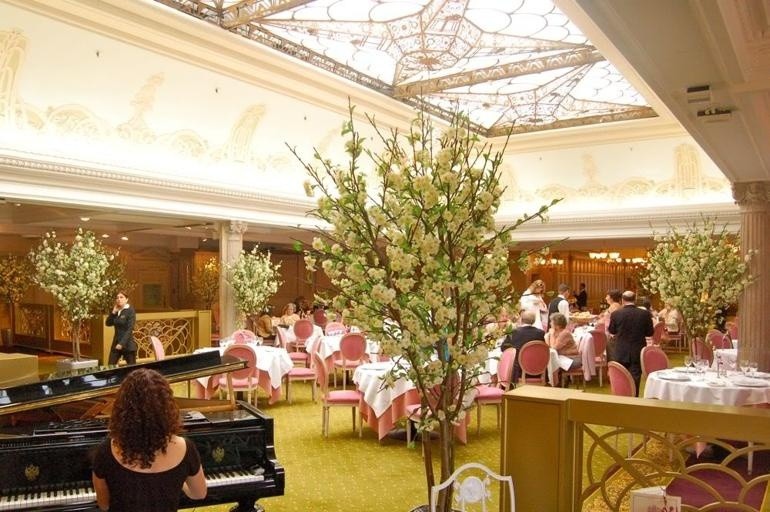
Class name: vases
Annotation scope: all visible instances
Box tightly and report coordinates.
[56,359,98,378]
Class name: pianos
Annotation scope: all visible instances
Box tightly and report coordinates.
[0,350,284,511]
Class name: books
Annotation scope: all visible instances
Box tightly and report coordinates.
[630,484,683,512]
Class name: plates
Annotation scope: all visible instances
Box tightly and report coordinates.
[746,372,770,378]
[734,379,768,387]
[659,374,690,380]
[674,367,698,373]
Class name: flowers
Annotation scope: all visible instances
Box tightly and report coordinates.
[219,240,286,336]
[635,207,760,359]
[281,75,568,512]
[0,252,38,344]
[192,254,220,310]
[26,225,136,361]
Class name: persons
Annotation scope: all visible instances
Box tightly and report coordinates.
[92,368,207,512]
[254,302,341,341]
[712,316,734,349]
[500,279,680,397]
[105,292,137,366]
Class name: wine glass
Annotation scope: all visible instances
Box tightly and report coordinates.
[219,335,264,351]
[327,329,345,337]
[684,354,709,380]
[728,355,758,380]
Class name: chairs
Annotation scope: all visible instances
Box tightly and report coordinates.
[429,463,517,512]
[150,300,740,455]
